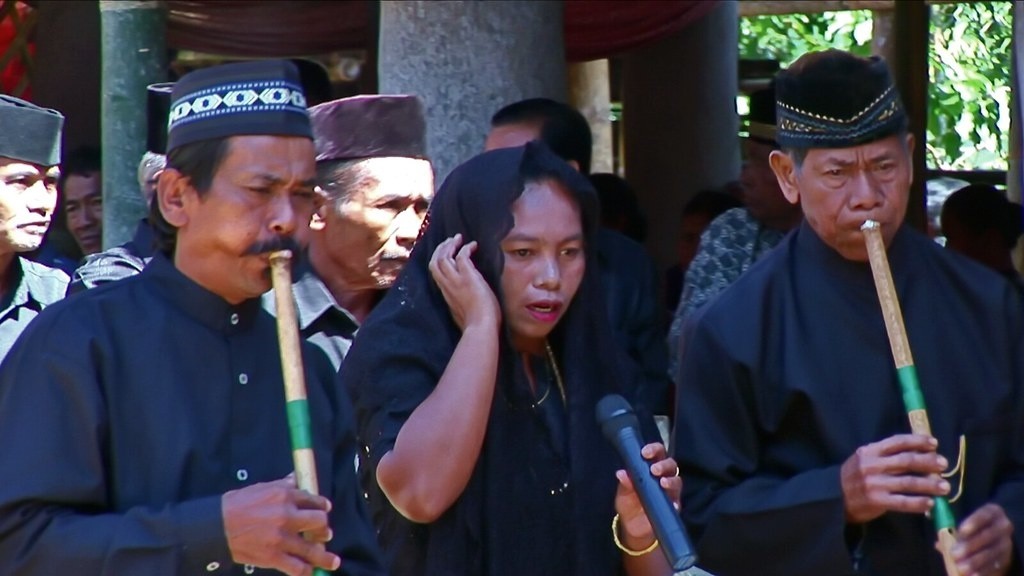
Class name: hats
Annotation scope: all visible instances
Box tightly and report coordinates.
[0,93,65,167]
[164,57,315,158]
[940,183,1024,251]
[772,46,907,148]
[307,94,431,164]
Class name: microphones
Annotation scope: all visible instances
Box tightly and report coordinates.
[596,392,698,573]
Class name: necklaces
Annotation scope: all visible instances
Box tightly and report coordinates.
[500,363,550,412]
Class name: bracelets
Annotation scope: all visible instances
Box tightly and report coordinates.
[612,514,658,556]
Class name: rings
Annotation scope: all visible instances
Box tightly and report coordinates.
[675,467,679,477]
[994,559,999,569]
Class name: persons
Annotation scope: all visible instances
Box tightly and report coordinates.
[0,48,1024,576]
